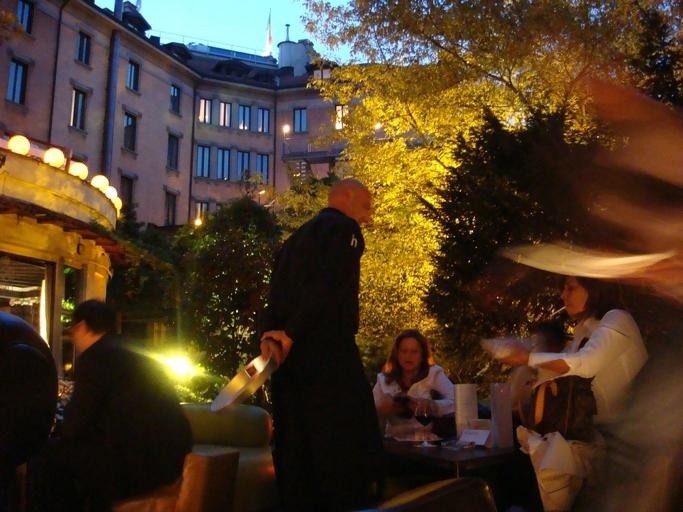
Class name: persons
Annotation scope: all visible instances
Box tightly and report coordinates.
[255,178,385,512]
[372,330,455,441]
[498,276,648,512]
[26,300,195,512]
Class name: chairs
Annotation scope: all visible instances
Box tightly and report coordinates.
[168,447,240,512]
[383,474,501,511]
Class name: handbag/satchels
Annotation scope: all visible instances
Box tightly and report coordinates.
[526,375,596,438]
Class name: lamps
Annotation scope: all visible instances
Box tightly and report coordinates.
[4,133,122,221]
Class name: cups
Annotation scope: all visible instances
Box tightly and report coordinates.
[452,382,478,438]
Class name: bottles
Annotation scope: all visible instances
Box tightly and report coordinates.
[488,380,516,450]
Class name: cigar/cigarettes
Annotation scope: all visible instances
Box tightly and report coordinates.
[552,306,566,316]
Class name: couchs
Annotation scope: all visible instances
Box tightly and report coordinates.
[176,397,275,512]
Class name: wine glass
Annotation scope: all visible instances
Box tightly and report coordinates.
[413,396,436,449]
